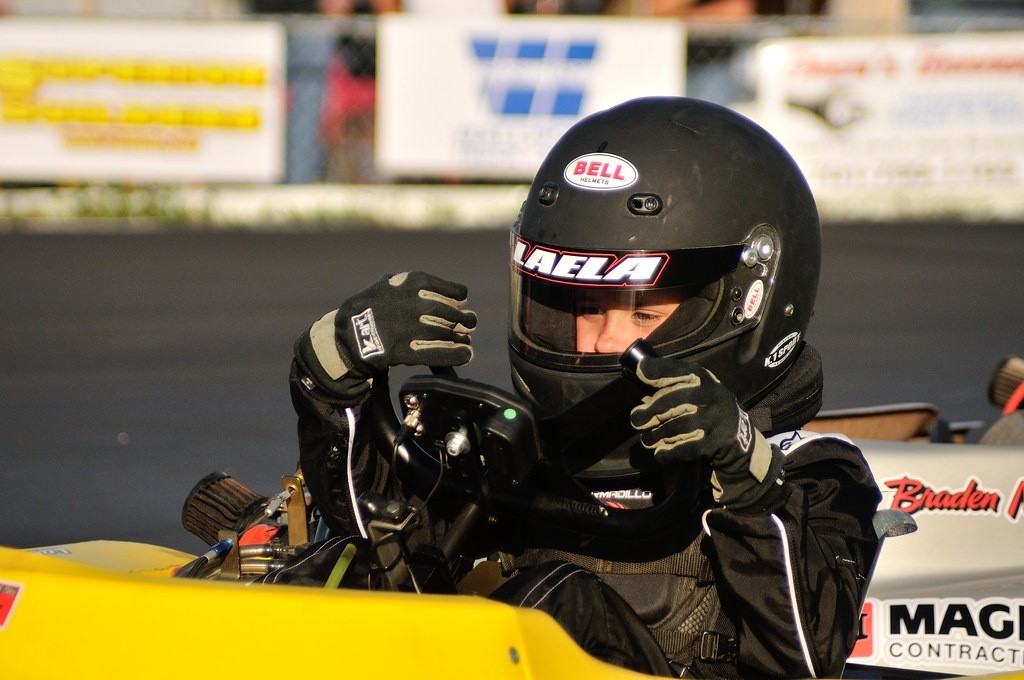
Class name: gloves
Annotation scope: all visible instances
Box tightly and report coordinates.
[292,269,479,406]
[629,356,787,512]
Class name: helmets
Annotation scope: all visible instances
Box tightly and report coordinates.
[508,96,823,479]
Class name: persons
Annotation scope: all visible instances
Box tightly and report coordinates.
[289,96,884,680]
[244,0,832,17]
